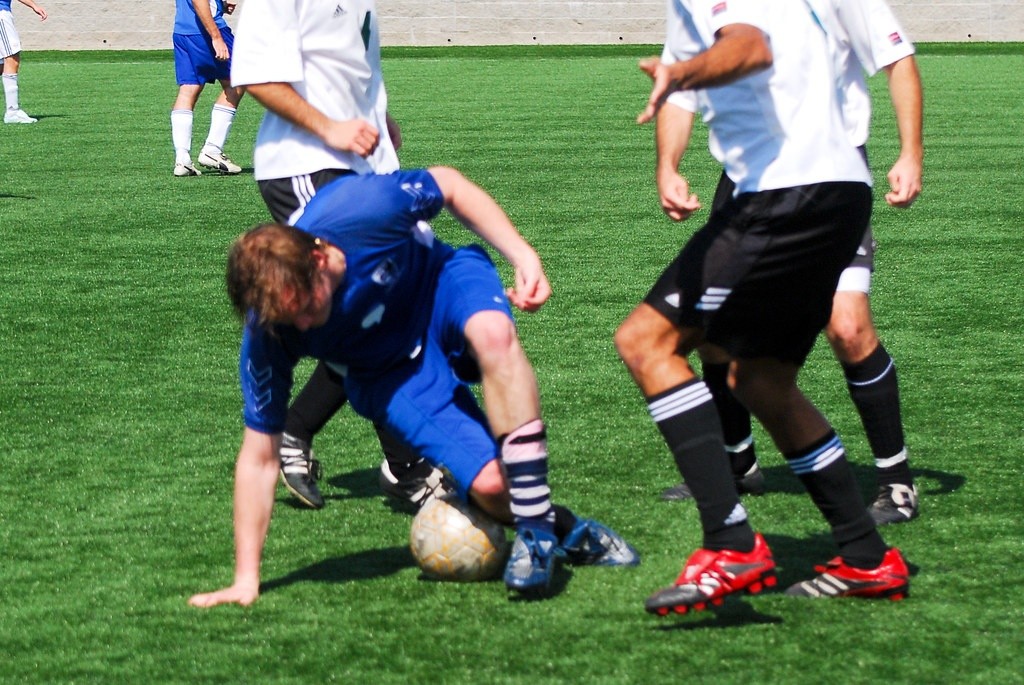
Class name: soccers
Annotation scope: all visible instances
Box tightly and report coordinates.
[408,492,508,583]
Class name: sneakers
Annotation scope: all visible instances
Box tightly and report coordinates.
[662,465,765,500]
[173,162,202,176]
[784,547,908,602]
[379,458,456,508]
[865,482,918,525]
[560,513,639,566]
[279,431,324,510]
[4,109,38,124]
[504,524,558,592]
[198,144,242,174]
[645,533,776,616]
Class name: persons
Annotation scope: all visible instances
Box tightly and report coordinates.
[171,0,243,178]
[616,0,909,615]
[655,0,923,523]
[187,165,637,608]
[0,0,47,123]
[230,0,443,505]
